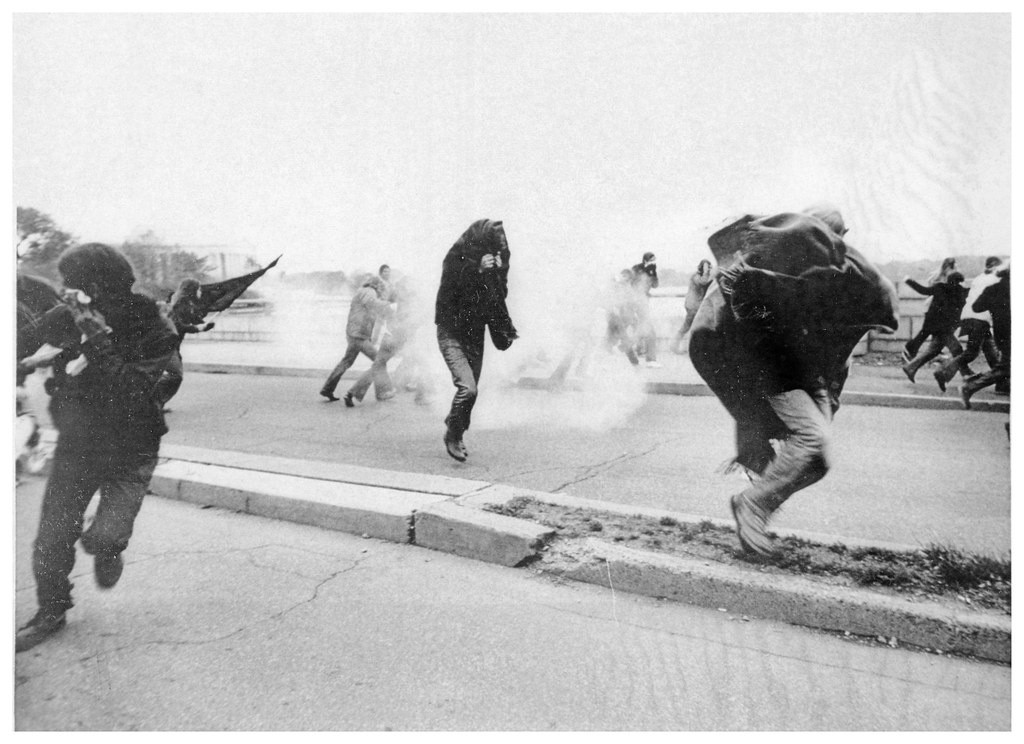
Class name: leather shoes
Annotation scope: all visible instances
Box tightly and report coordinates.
[443,431,469,462]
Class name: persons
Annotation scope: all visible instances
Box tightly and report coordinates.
[15,243,216,653]
[320,207,1011,559]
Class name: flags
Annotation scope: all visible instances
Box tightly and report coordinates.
[167,258,279,313]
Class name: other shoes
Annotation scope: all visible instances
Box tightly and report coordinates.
[321,388,339,401]
[958,383,973,409]
[96,551,123,587]
[995,387,1011,398]
[902,364,918,384]
[344,393,355,409]
[934,369,949,394]
[731,493,776,559]
[16,608,67,653]
[900,348,913,364]
[377,386,398,401]
